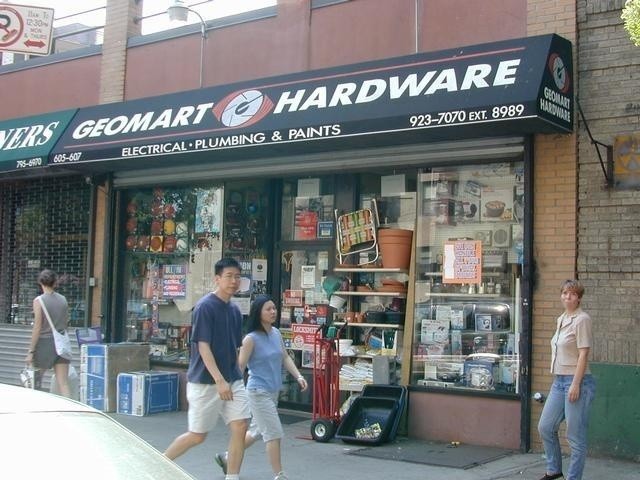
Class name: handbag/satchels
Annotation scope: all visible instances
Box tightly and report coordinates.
[52,329,73,360]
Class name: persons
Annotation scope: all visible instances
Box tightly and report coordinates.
[214,293,309,480]
[536,278,599,480]
[161,256,253,480]
[23,268,74,399]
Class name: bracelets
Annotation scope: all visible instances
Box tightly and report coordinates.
[27,349,35,354]
[295,376,304,380]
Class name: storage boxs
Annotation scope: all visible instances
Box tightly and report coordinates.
[117,370,179,416]
[79,341,150,412]
[360,384,407,442]
[335,396,398,445]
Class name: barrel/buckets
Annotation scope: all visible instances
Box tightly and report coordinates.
[377,228,413,268]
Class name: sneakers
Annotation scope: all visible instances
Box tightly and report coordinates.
[215,452,229,474]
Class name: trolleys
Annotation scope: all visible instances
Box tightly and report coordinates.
[305,321,349,439]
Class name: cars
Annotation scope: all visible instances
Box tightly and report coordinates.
[0,381,196,480]
[408,298,527,383]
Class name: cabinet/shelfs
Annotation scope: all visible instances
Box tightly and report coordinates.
[329,267,409,390]
[412,271,505,364]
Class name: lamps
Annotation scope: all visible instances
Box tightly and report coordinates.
[166,0,205,88]
